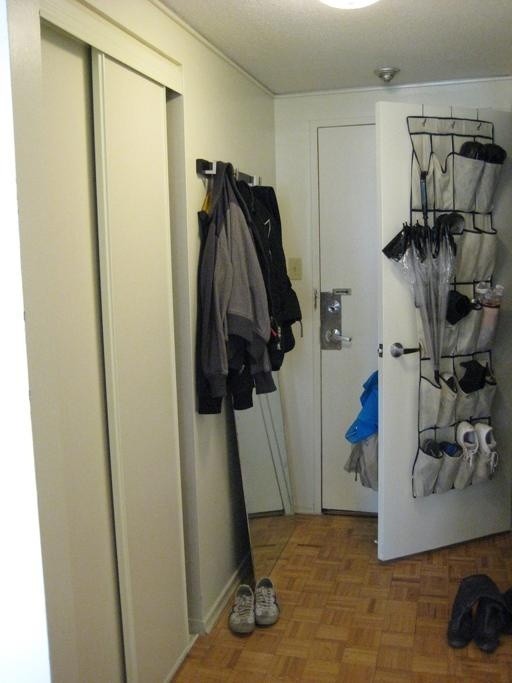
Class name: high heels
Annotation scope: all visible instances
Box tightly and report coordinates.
[460,360,487,420]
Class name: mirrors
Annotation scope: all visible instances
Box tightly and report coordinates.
[231,369,297,589]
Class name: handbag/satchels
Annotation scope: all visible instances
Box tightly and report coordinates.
[338,437,378,490]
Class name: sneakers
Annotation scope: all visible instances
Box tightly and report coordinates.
[435,440,462,495]
[436,372,458,428]
[453,421,480,490]
[228,583,255,634]
[254,577,280,625]
[474,423,498,488]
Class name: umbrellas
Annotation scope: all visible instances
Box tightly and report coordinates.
[379,170,460,385]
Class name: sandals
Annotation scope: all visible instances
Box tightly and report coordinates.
[414,439,442,494]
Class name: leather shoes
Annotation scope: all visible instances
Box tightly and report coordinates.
[485,143,507,164]
[459,141,488,161]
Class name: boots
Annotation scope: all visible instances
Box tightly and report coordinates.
[446,574,512,650]
[472,586,512,655]
